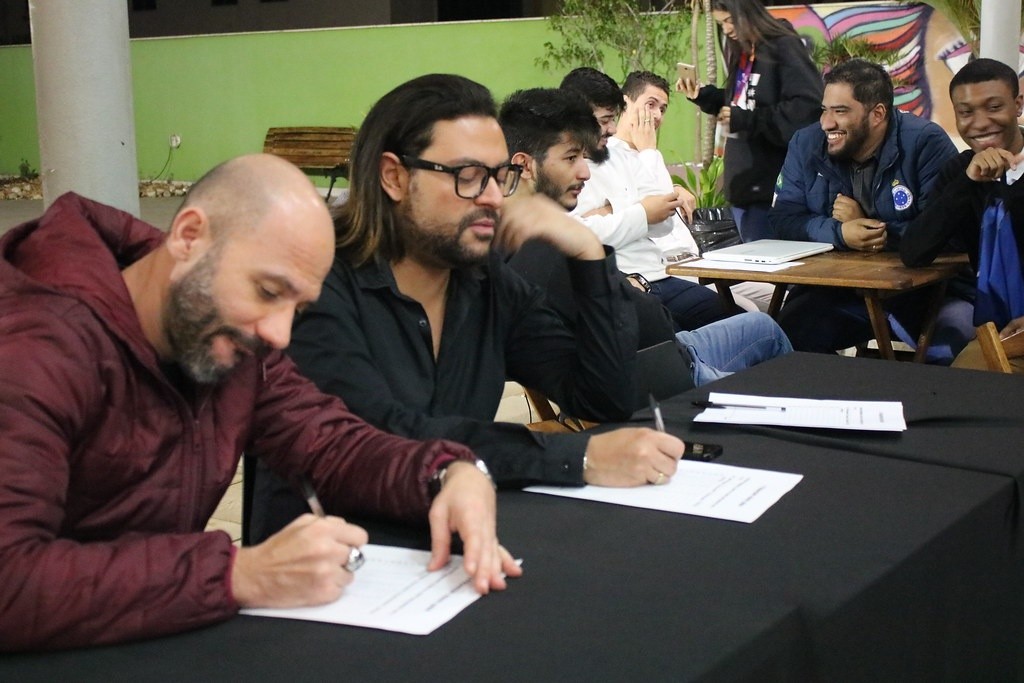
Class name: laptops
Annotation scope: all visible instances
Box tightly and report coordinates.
[702,239,834,265]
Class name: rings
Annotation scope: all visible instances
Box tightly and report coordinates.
[343,547,364,571]
[655,473,664,484]
[873,245,875,250]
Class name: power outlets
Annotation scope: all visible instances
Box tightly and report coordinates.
[169,132,181,150]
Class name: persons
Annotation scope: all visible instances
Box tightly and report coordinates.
[0,155,522,657]
[899,58,1024,367]
[240,72,683,547]
[676,0,826,242]
[767,59,978,367]
[497,67,792,401]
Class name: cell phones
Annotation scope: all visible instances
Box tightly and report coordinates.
[681,441,723,462]
[677,63,696,91]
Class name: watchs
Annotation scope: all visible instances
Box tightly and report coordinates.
[430,453,499,498]
[625,273,653,293]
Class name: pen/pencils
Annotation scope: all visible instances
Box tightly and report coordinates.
[690,401,786,412]
[649,393,666,432]
[301,479,325,517]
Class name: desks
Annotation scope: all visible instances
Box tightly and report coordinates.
[0,351,1024,683]
[666,236,966,362]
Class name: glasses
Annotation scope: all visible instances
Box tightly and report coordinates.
[399,154,523,200]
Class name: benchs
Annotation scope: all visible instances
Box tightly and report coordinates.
[257,125,360,209]
